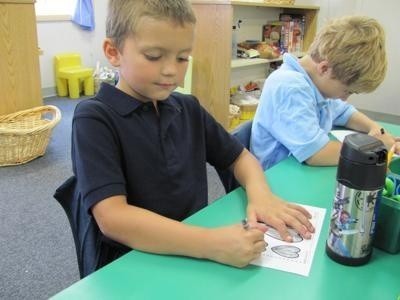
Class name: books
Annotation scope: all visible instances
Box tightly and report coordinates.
[236,12,305,60]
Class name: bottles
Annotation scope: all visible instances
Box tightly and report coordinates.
[324,132,388,268]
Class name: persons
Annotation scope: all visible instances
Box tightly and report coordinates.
[249,14,400,171]
[70,0,315,270]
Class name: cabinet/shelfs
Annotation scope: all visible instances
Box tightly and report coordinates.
[190,0,320,134]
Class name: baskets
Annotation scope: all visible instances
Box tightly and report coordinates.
[0,105,62,167]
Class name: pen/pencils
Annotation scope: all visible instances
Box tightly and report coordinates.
[243,220,248,229]
[387,145,395,167]
[381,128,384,134]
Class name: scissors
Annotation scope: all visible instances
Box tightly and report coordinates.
[382,174,400,202]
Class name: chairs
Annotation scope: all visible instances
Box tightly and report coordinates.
[231,120,252,190]
[51,173,87,281]
[55,53,94,99]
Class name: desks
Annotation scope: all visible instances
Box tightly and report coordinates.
[49,120,400,300]
